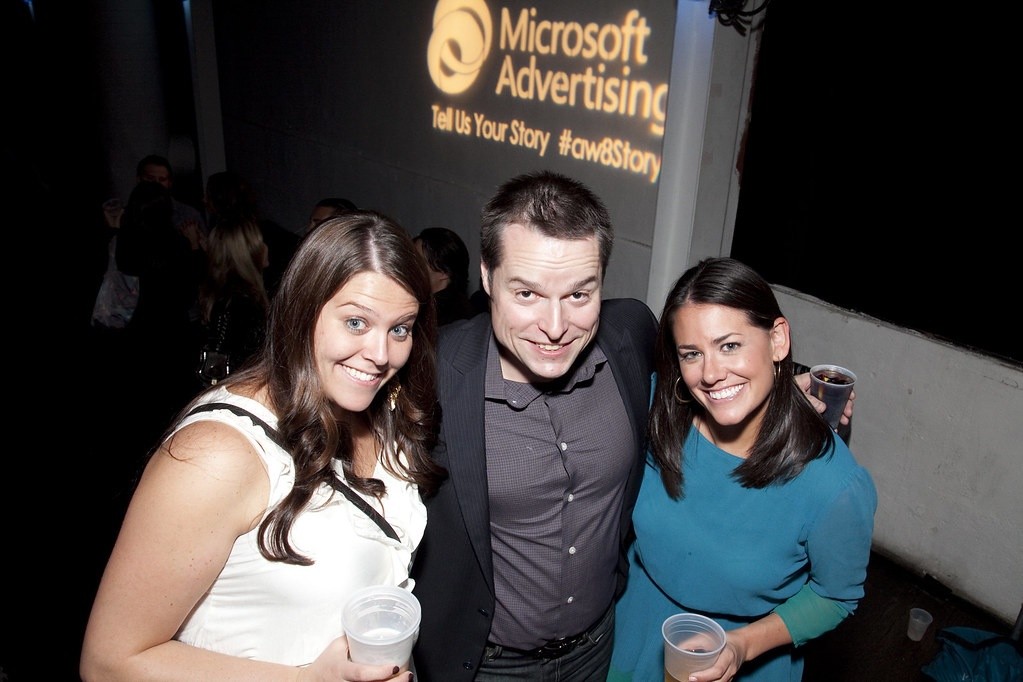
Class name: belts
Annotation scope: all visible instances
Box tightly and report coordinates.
[484,597,620,659]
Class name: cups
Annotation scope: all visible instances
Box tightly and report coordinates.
[339,585,421,681]
[662,611,727,682]
[103,199,122,219]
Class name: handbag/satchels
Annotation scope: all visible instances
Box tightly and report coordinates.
[188,306,232,389]
[91,235,140,329]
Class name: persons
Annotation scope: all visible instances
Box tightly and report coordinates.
[606,257,878,682]
[398,172,855,682]
[104,153,475,401]
[79,212,432,682]
